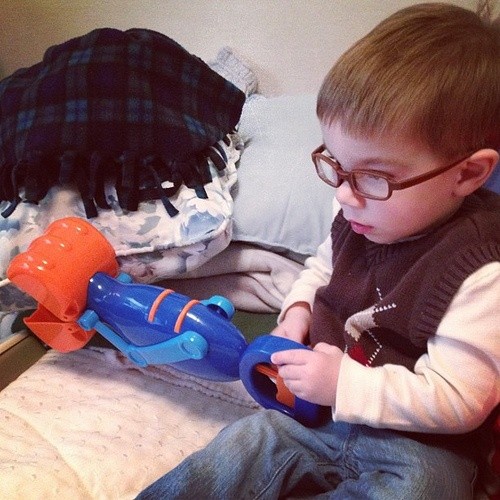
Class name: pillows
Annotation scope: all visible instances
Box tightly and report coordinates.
[1,130,243,312]
[229,93,340,266]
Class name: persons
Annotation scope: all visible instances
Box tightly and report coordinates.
[130,0,500,500]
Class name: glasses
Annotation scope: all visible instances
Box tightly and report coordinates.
[310,143,475,202]
